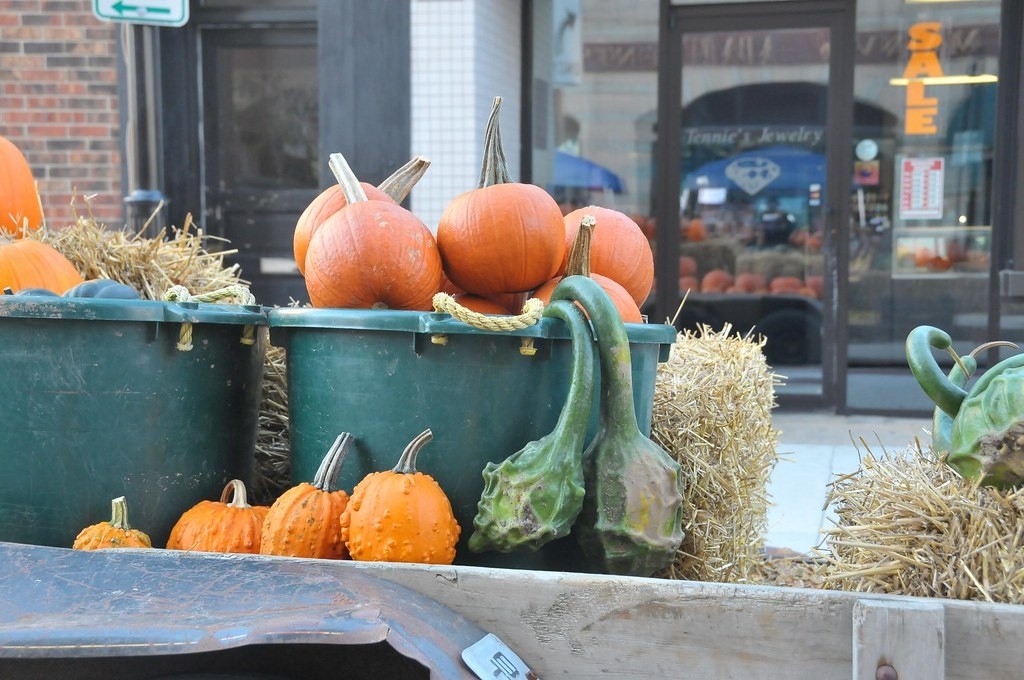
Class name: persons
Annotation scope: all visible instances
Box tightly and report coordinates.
[732,195,797,248]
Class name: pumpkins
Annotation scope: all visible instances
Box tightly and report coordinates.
[0,95,656,583]
[632,213,992,295]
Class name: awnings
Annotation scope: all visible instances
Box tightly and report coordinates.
[554,149,623,195]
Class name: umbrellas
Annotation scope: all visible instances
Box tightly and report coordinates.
[681,146,859,191]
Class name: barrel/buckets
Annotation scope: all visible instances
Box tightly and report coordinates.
[0,294,268,551]
[266,307,678,571]
